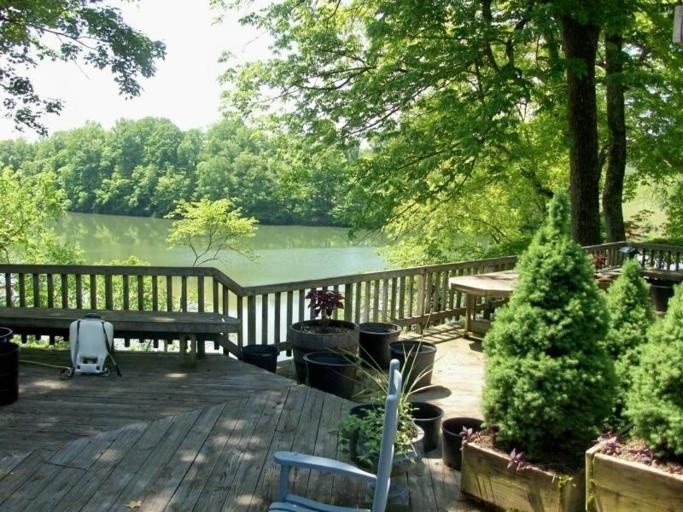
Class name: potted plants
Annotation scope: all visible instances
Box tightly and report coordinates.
[287,288,360,381]
[457,189,683,511]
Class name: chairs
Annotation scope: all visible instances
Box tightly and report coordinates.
[268,358,403,512]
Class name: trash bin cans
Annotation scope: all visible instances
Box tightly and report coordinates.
[0,327,19,406]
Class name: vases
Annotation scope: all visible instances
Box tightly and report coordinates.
[303,320,485,470]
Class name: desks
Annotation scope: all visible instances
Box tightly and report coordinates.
[449,269,520,341]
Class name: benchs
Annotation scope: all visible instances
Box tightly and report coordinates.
[1,310,241,369]
